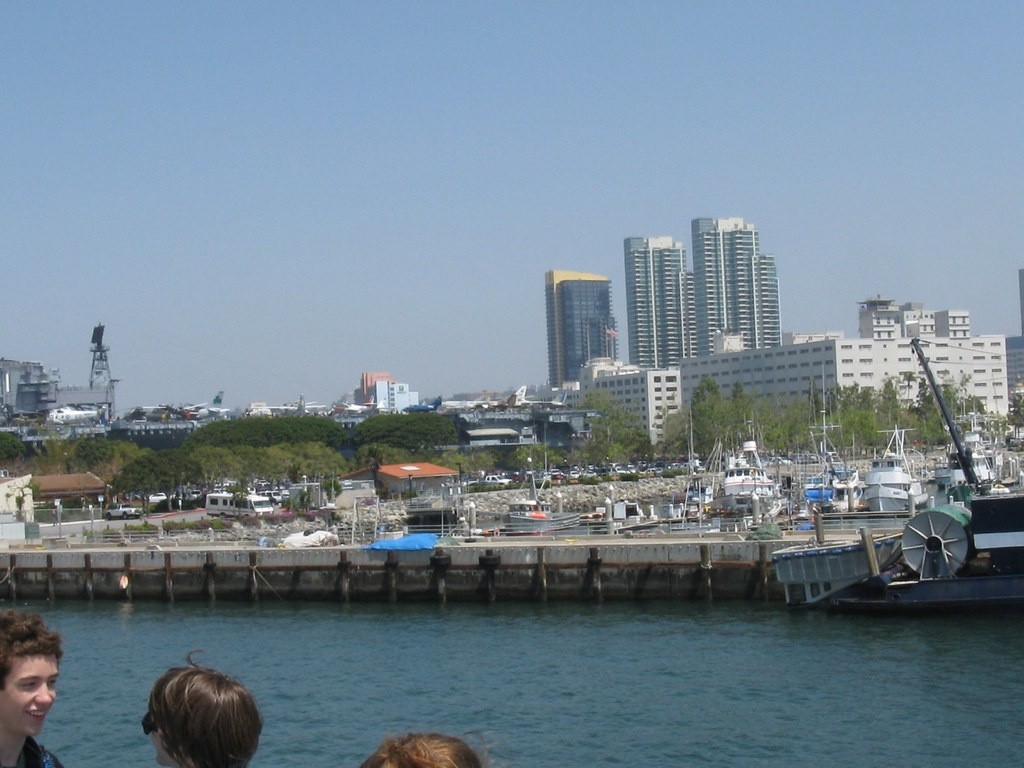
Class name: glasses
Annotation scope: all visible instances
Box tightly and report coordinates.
[141,710,159,735]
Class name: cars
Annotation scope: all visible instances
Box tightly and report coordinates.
[124,473,335,506]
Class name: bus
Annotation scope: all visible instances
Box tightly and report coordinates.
[204,492,274,519]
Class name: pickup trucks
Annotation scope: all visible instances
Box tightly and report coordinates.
[106,502,143,520]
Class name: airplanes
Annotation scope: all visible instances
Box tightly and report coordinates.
[46,392,446,425]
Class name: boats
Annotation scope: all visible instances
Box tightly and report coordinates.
[452,404,1024,531]
[769,335,1023,612]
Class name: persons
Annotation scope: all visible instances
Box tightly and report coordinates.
[358,732,482,768]
[141,649,264,768]
[0,609,65,768]
